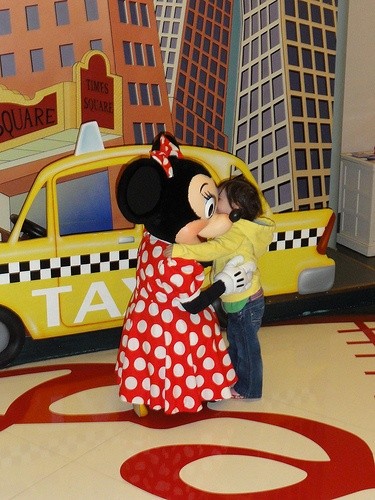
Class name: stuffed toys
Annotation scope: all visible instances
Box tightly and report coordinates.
[112,132,255,417]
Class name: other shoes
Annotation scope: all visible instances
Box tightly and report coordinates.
[229,388,262,399]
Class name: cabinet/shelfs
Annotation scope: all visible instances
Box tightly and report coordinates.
[337,153,375,257]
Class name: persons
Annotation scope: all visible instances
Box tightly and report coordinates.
[163,175,276,401]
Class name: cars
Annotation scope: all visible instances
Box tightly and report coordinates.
[0,119,338,373]
